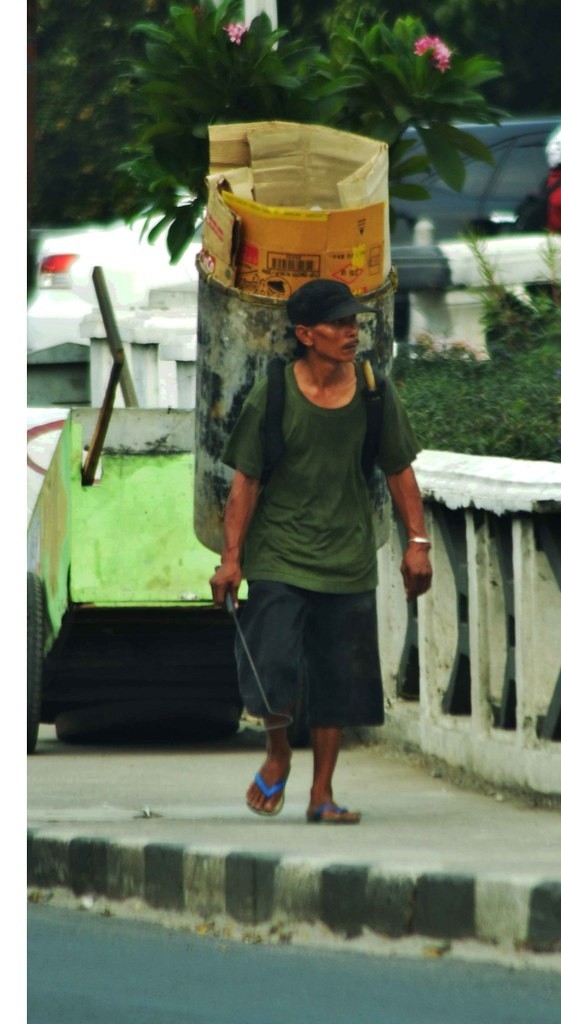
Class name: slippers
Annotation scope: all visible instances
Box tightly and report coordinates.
[307,802,360,824]
[246,756,292,817]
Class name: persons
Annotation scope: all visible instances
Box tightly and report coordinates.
[209,279,436,824]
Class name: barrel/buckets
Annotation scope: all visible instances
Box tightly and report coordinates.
[195,251,401,562]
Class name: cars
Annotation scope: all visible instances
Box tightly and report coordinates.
[389,117,561,242]
[27,192,203,350]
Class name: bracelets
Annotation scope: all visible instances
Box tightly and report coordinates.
[407,536,432,546]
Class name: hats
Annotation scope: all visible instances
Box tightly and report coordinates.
[287,280,381,325]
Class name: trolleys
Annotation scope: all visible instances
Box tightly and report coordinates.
[28,353,315,752]
